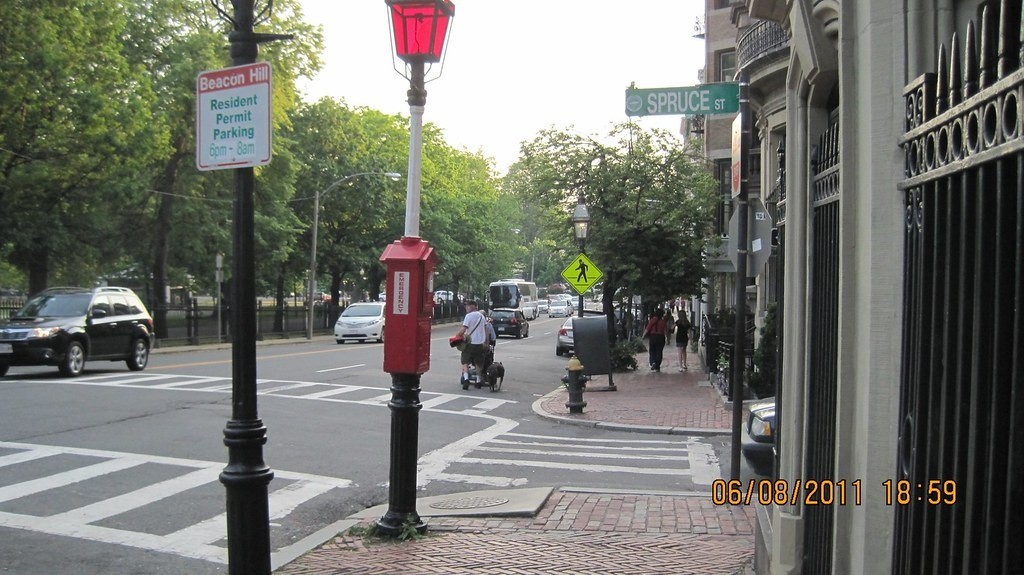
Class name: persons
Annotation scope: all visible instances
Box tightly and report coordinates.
[642,297,692,373]
[456,300,489,390]
[478,309,496,340]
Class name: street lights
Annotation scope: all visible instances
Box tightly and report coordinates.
[569,199,590,318]
[375,0,456,540]
[304,171,402,341]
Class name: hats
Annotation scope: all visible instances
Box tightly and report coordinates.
[464,299,477,306]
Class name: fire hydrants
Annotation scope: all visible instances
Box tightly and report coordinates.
[560,355,590,414]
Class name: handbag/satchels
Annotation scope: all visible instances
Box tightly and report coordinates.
[458,336,471,352]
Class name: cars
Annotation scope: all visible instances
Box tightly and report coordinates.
[432,290,464,305]
[741,395,775,478]
[536,282,631,319]
[485,307,530,339]
[334,301,387,344]
[555,315,593,357]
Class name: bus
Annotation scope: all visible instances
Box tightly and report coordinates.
[483,278,539,322]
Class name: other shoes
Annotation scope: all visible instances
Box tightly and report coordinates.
[462,379,469,390]
[651,363,656,370]
[656,367,660,372]
[475,382,481,389]
[680,369,683,372]
[684,366,687,370]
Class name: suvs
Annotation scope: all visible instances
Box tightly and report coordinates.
[0,284,157,379]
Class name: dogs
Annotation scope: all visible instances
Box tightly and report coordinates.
[486,362,505,392]
